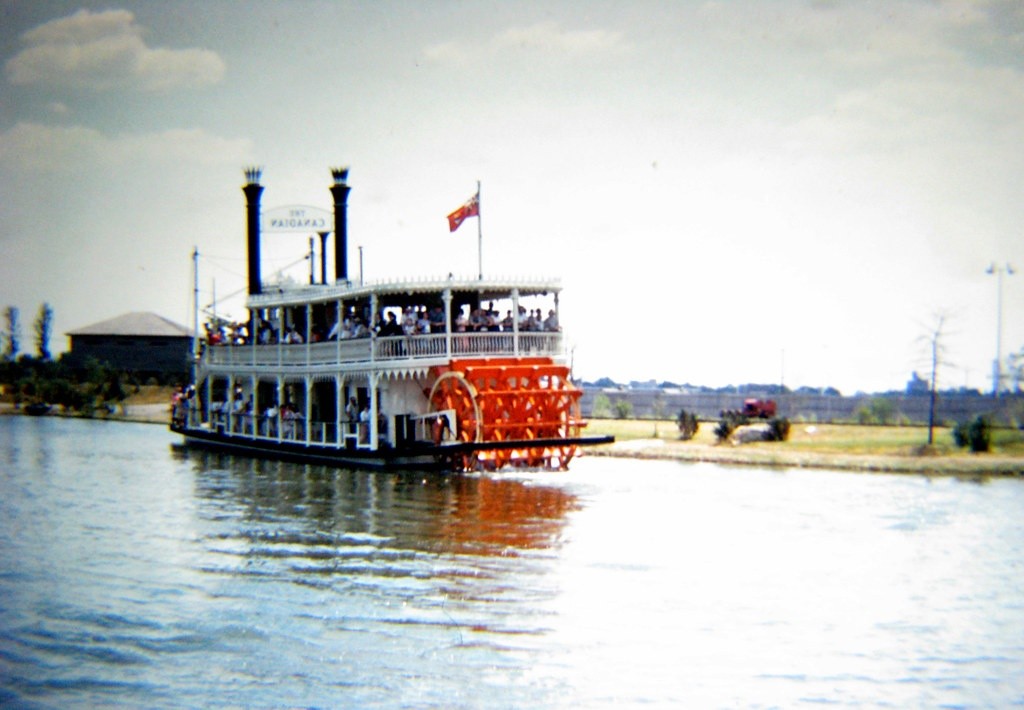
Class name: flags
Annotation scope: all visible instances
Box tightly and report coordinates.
[447,191,482,232]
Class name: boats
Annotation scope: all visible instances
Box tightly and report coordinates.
[170,159,615,475]
[25,400,52,417]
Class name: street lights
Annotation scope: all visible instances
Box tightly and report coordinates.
[985,264,1014,370]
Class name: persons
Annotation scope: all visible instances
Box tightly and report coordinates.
[172,302,560,445]
